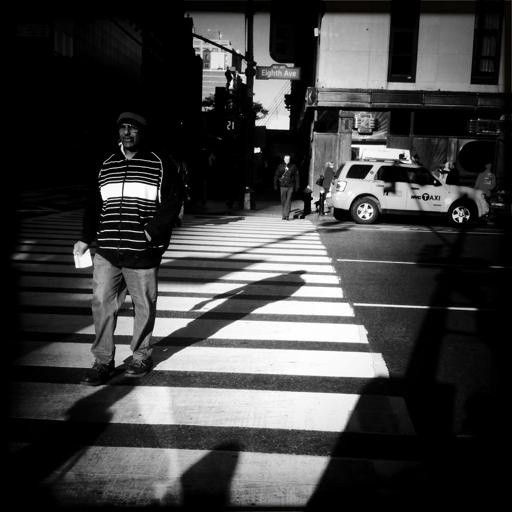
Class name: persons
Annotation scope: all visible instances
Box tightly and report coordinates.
[272,153,300,221]
[472,159,497,203]
[78,112,184,386]
[314,160,336,216]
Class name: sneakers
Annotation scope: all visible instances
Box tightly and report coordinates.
[80,357,117,388]
[123,355,154,379]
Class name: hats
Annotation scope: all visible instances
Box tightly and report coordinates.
[116,112,153,129]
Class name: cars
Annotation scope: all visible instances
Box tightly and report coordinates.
[331,160,494,229]
